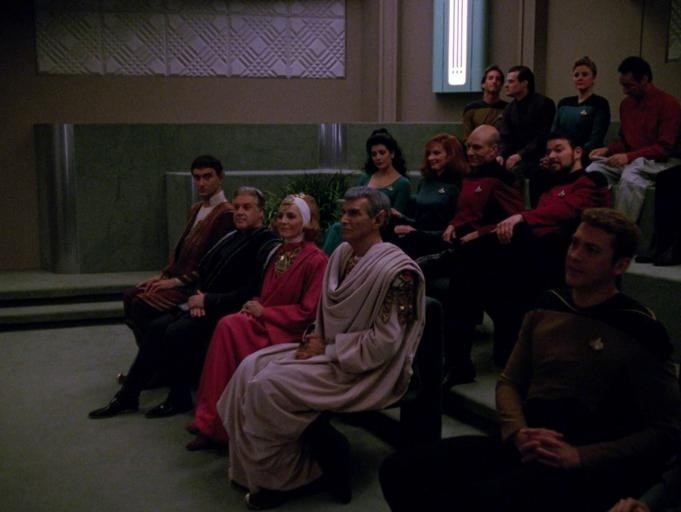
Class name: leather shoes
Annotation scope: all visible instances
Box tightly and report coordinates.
[441,358,477,383]
[88,398,140,420]
[656,247,679,266]
[635,249,657,263]
[142,396,194,419]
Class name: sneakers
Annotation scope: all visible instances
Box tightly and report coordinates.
[244,490,297,509]
[230,479,250,491]
[186,437,211,451]
[188,422,202,434]
[117,373,127,384]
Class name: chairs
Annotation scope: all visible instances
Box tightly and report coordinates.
[604,119,670,265]
[291,295,447,456]
[481,289,527,356]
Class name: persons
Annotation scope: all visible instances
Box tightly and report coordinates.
[463,57,681,266]
[90,154,425,511]
[320,124,681,512]
